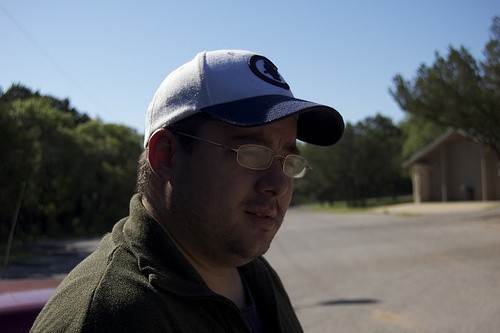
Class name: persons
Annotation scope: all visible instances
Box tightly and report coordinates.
[27,46,348,333]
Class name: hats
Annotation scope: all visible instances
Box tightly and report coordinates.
[143,49,344,146]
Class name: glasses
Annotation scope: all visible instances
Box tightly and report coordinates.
[169,126,312,178]
